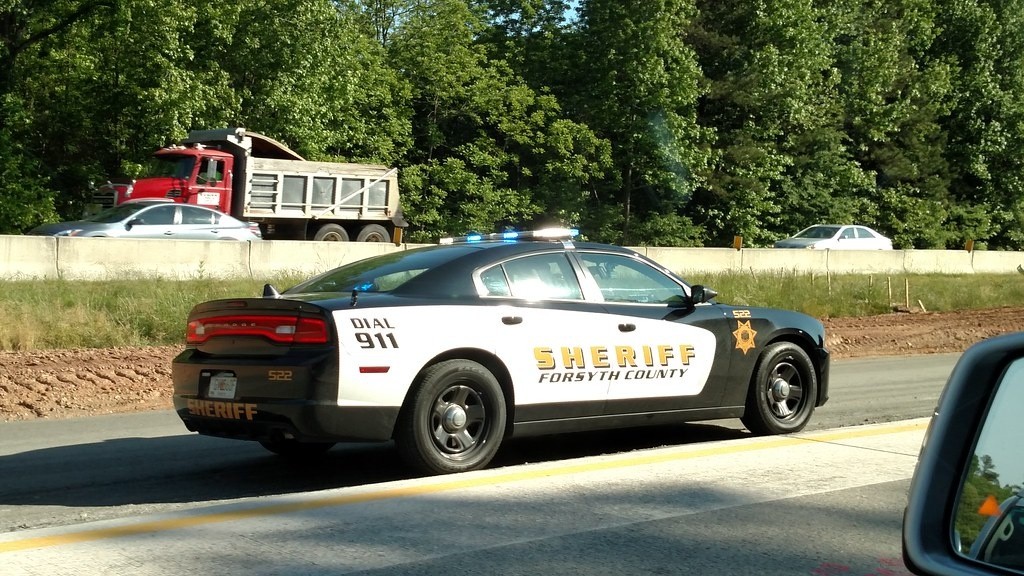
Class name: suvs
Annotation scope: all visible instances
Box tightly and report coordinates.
[969,486,1024,574]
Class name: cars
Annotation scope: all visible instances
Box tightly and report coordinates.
[772,223,893,251]
[172,226,829,473]
[24,196,264,240]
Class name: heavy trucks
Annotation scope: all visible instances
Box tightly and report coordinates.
[86,126,410,242]
